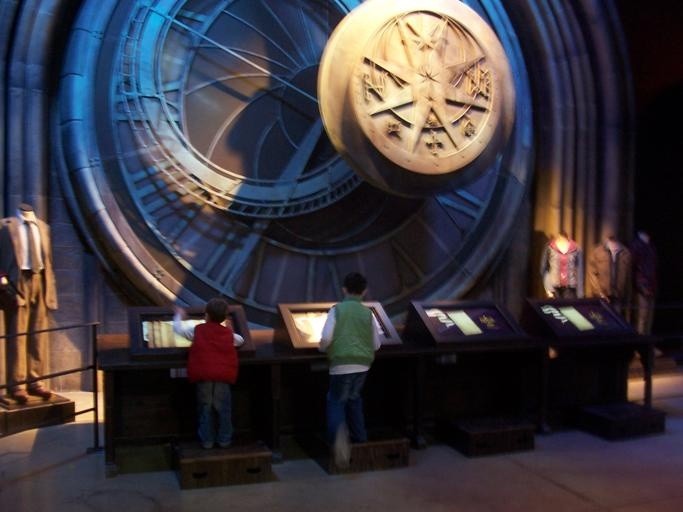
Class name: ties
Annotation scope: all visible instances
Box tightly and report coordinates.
[23,220,40,274]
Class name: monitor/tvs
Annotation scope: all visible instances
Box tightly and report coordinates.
[126,305,258,356]
[528,296,636,342]
[276,299,402,353]
[408,296,528,348]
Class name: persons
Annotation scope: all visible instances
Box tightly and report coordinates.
[541,231,584,301]
[172,299,245,450]
[633,231,663,357]
[585,235,631,326]
[0,203,58,400]
[319,272,384,473]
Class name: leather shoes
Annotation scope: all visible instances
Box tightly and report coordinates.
[30,387,53,397]
[12,390,29,402]
